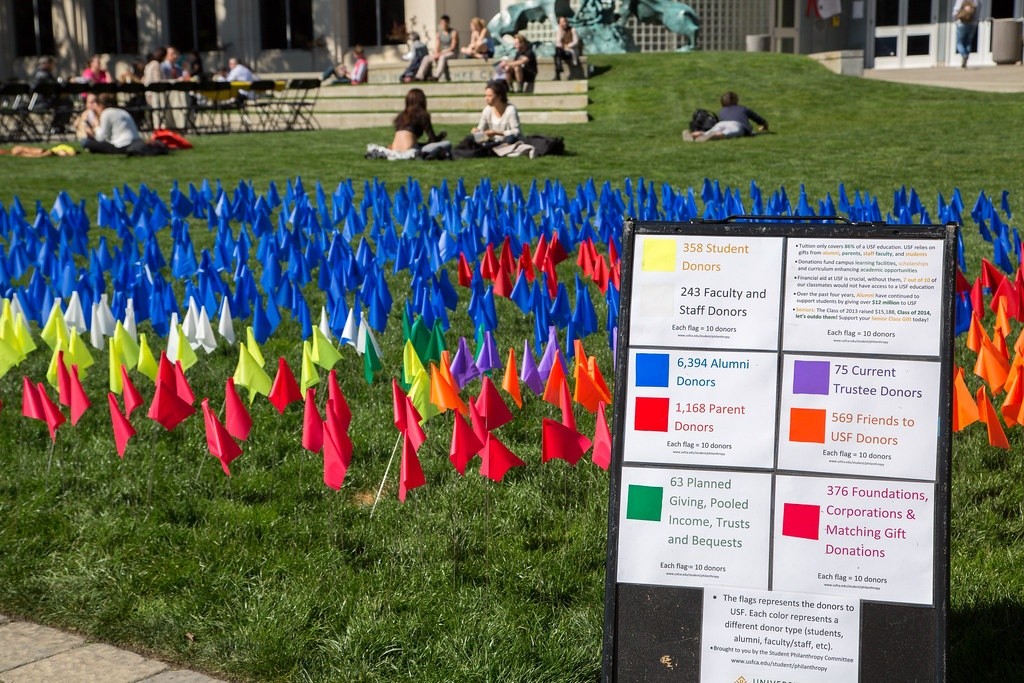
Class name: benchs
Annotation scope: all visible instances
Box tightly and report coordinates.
[810,50,866,77]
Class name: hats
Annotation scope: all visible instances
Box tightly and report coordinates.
[487,79,510,94]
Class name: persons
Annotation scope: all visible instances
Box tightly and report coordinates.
[682,91,768,143]
[460,17,495,58]
[391,88,447,153]
[470,78,523,156]
[319,45,368,89]
[399,14,458,83]
[499,35,537,94]
[552,16,580,82]
[17,45,261,154]
[951,0,980,69]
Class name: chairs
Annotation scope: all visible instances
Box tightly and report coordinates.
[0,73,322,147]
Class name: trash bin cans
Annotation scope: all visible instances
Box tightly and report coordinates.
[746,35,770,53]
[992,18,1022,66]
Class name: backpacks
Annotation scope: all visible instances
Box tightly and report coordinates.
[524,135,567,156]
[689,108,719,131]
[126,138,169,156]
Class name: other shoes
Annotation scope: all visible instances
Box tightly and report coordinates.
[428,76,439,82]
[682,129,694,143]
[516,81,523,92]
[507,83,514,93]
[694,131,715,143]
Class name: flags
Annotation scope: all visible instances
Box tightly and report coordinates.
[0,174,1024,505]
